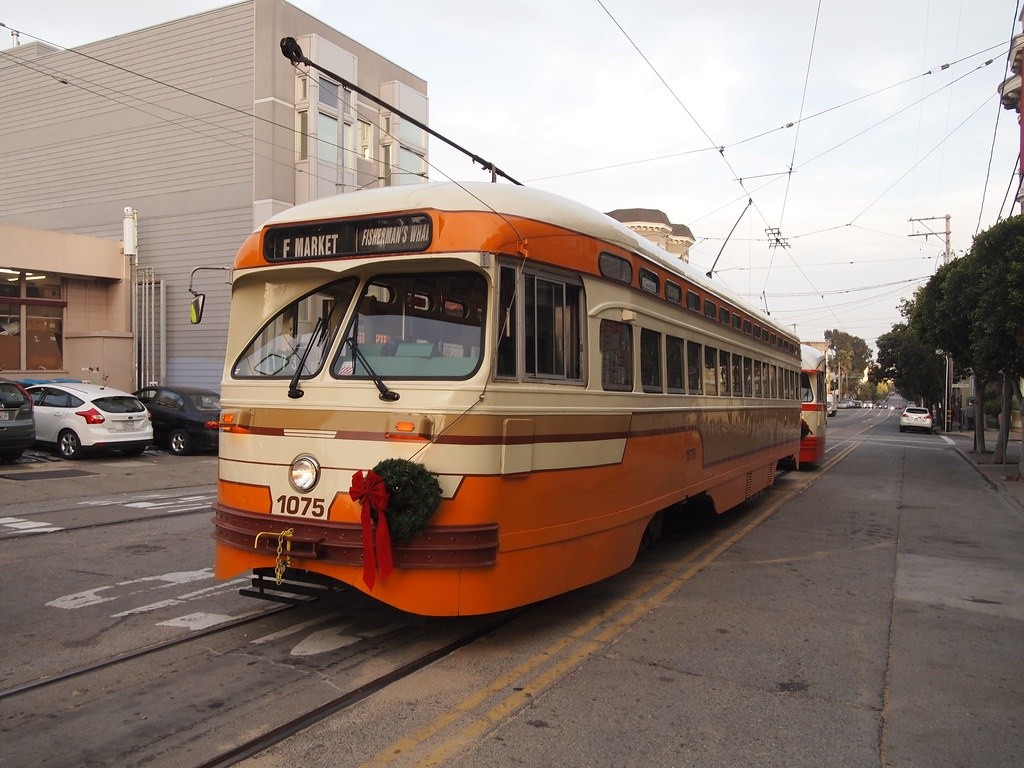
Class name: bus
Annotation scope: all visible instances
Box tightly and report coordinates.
[189,181,827,617]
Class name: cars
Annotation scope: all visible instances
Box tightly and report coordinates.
[827,396,916,417]
[899,407,932,434]
[0,378,220,460]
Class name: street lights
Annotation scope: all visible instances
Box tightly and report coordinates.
[935,349,948,432]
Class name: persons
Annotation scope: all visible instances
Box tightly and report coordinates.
[965,403,975,431]
